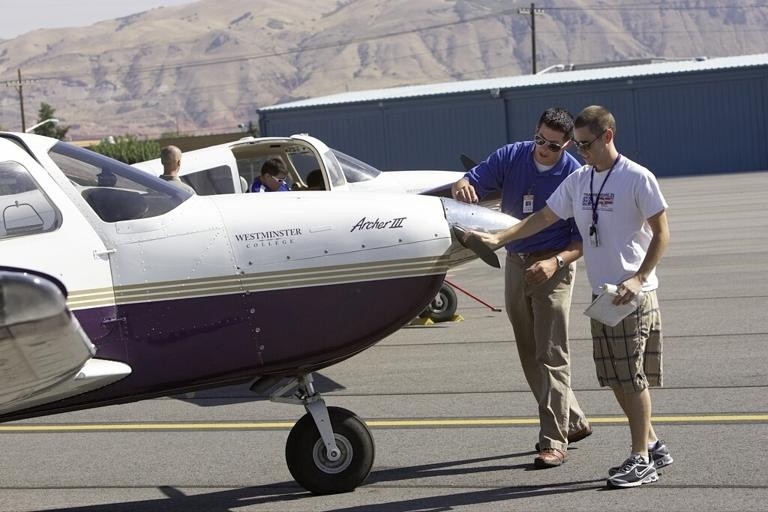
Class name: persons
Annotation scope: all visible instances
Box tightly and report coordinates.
[249,157,292,192]
[463,105,674,488]
[451,107,583,467]
[159,145,197,195]
[298,169,325,190]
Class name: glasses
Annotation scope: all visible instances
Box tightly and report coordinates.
[571,129,607,150]
[270,176,286,183]
[534,130,572,153]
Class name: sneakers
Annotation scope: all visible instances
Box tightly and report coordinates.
[607,440,675,490]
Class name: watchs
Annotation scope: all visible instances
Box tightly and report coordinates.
[555,255,566,273]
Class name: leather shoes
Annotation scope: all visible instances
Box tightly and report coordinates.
[534,424,593,467]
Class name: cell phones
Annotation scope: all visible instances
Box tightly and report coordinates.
[588,224,600,247]
